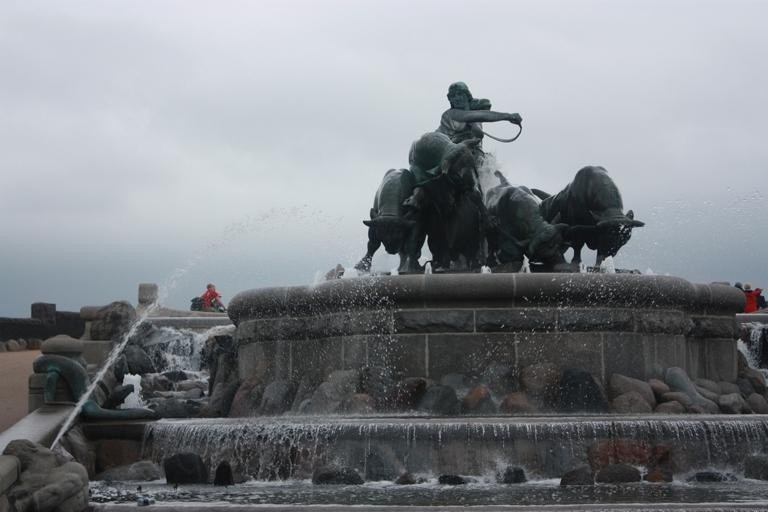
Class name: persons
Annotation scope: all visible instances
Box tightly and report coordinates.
[734,282,766,313]
[436,82,522,152]
[200,283,226,309]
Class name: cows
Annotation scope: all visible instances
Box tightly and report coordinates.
[353,131,647,275]
